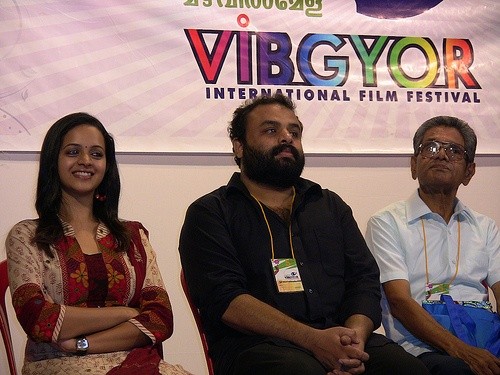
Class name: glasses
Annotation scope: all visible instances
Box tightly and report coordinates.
[416,140,470,160]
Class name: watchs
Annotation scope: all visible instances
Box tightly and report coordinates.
[75,334,90,356]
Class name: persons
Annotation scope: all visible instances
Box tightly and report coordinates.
[178,93,430,375]
[6,113,191,375]
[364,116,500,375]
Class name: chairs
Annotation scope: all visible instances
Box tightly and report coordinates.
[180,268,215,375]
[0,259,21,375]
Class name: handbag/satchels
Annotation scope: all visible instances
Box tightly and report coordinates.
[420,295,500,359]
